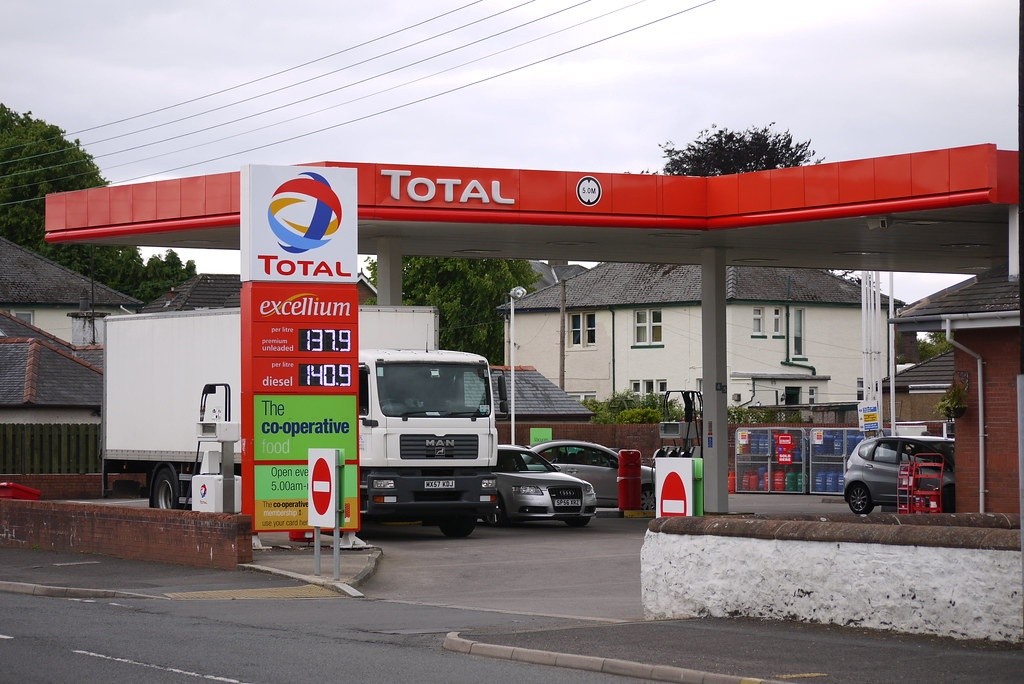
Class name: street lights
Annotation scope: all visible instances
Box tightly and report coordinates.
[510,286,526,446]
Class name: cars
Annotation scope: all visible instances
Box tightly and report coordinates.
[843,436,957,515]
[520,440,658,511]
[479,445,598,528]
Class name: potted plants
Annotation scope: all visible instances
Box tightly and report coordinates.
[932,384,969,422]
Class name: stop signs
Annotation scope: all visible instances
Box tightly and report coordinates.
[312,457,332,515]
[661,471,687,519]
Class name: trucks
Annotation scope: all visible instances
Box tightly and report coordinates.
[104,305,509,538]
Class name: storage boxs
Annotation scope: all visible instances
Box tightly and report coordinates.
[806,425,891,496]
[734,424,809,494]
